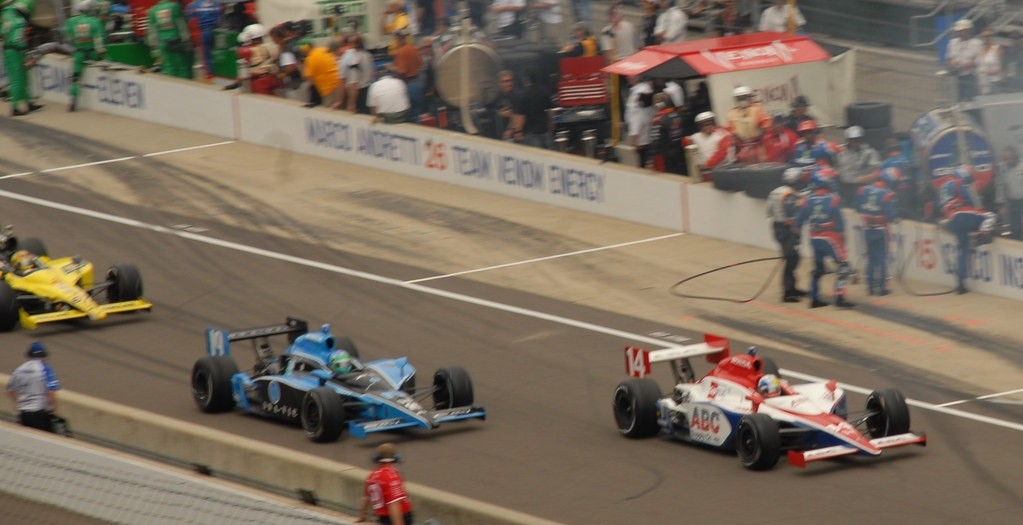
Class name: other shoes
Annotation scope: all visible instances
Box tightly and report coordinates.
[14,109,27,116]
[778,285,969,309]
[29,104,44,112]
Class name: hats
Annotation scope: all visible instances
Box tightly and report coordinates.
[27,342,47,356]
[954,18,973,32]
[789,95,810,106]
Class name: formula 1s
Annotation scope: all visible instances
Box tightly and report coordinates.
[612,333,929,472]
[189,313,488,444]
[0,222,155,334]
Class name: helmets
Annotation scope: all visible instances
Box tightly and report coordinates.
[797,120,816,131]
[15,3,28,15]
[954,168,972,183]
[280,52,298,68]
[10,249,33,271]
[845,125,865,138]
[244,23,267,41]
[695,112,714,122]
[758,373,782,397]
[884,167,901,187]
[328,350,351,374]
[811,168,841,189]
[78,1,92,11]
[734,86,751,98]
[880,138,903,152]
[783,168,804,184]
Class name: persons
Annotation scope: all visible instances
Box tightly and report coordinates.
[7,342,70,436]
[11,250,36,272]
[0,0,1023,308]
[328,349,353,373]
[758,375,782,397]
[355,443,414,525]
